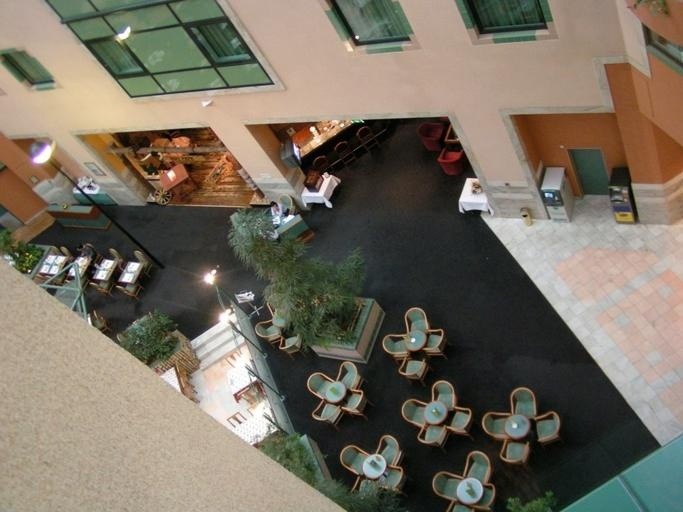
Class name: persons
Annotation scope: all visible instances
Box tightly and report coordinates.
[77,244,97,263]
[270,201,289,218]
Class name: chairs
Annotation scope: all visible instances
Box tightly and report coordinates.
[482,411,511,440]
[377,465,413,498]
[511,387,538,419]
[402,398,429,428]
[404,308,431,335]
[463,451,492,484]
[447,408,478,442]
[341,445,370,476]
[422,329,449,359]
[535,412,561,446]
[448,501,473,512]
[341,388,374,420]
[415,117,465,176]
[337,362,369,391]
[312,126,383,178]
[256,301,286,350]
[280,333,303,359]
[470,484,496,512]
[382,333,412,365]
[313,400,346,432]
[352,476,377,499]
[35,244,158,332]
[431,381,458,414]
[374,433,412,472]
[398,357,436,392]
[277,193,301,216]
[226,353,266,429]
[306,373,336,400]
[417,424,453,454]
[432,471,465,502]
[500,438,529,472]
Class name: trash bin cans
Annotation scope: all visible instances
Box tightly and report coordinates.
[520,208,532,226]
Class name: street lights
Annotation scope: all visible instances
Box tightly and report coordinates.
[28,141,164,269]
[200,268,251,316]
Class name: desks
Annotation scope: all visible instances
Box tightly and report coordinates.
[231,406,279,449]
[301,175,341,208]
[459,178,492,215]
[456,477,484,505]
[503,414,533,443]
[324,383,349,406]
[405,331,428,357]
[362,455,389,480]
[423,401,449,425]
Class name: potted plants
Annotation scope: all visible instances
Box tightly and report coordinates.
[225,207,386,366]
